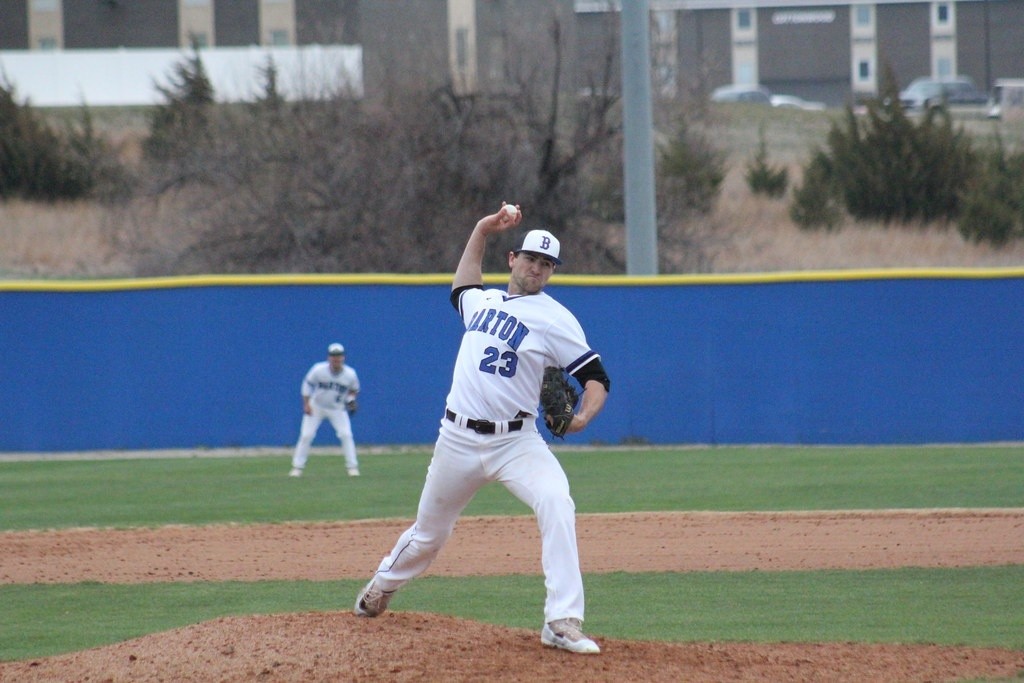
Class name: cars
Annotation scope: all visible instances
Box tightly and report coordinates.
[708,83,773,108]
[770,94,826,113]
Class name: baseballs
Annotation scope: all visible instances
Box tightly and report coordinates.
[501,204,518,222]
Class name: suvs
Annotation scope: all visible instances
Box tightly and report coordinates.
[882,75,978,111]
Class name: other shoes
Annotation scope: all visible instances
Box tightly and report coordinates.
[288,467,304,478]
[347,468,360,476]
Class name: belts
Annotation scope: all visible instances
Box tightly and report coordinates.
[446,408,522,434]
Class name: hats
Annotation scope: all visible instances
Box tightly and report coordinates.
[512,229,564,267]
[328,342,345,356]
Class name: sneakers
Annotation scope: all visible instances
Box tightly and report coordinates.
[354,578,392,616]
[541,618,601,655]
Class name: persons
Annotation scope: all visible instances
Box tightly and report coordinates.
[289,343,361,477]
[353,202,612,653]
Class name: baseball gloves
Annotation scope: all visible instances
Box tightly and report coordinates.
[538,364,588,444]
[347,399,358,416]
